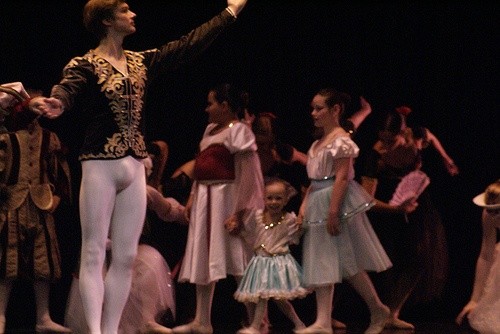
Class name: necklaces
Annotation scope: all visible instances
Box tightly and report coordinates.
[264,215,283,229]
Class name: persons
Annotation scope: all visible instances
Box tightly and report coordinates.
[0,0,264,334]
[227,90,500,334]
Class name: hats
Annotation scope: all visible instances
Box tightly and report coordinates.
[472,180,500,209]
